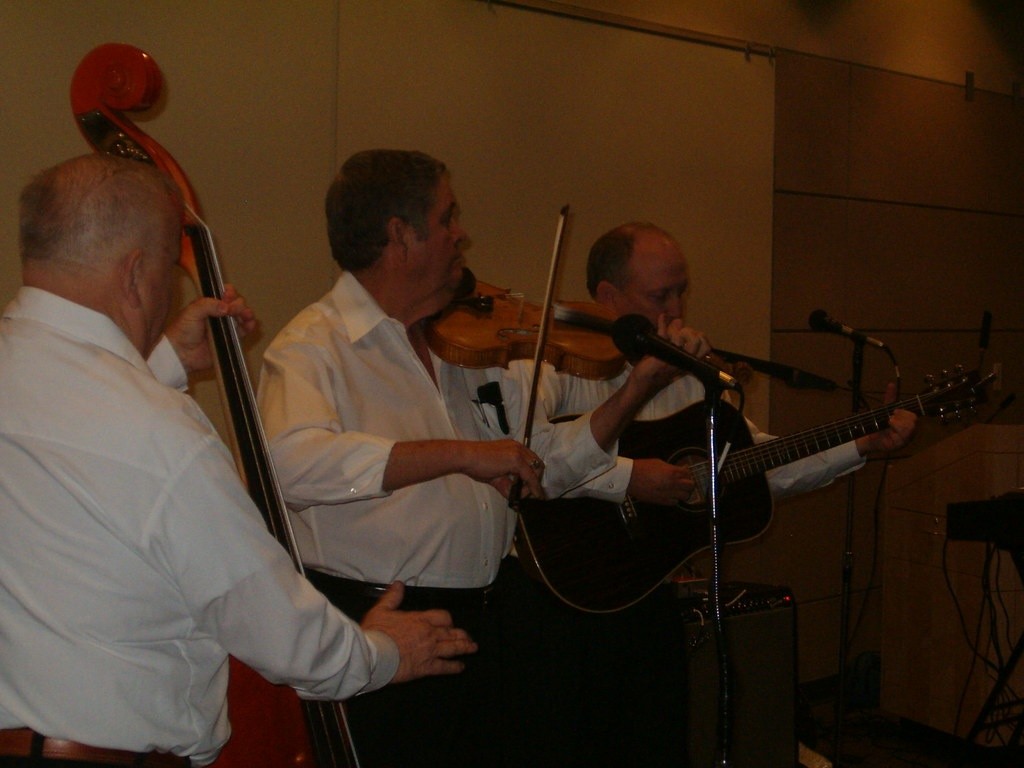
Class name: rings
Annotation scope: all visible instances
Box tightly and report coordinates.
[529,453,546,471]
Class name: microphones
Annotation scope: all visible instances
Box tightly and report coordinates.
[808,310,883,348]
[611,313,735,391]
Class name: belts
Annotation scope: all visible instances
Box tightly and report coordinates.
[0,728,193,767]
[304,569,517,609]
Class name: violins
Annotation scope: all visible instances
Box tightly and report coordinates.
[424,279,755,389]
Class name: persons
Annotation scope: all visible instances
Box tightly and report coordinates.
[499,218,917,578]
[252,140,715,768]
[0,150,482,768]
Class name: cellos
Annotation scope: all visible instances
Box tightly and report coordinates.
[66,45,366,768]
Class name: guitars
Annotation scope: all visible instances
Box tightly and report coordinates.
[509,362,1000,619]
